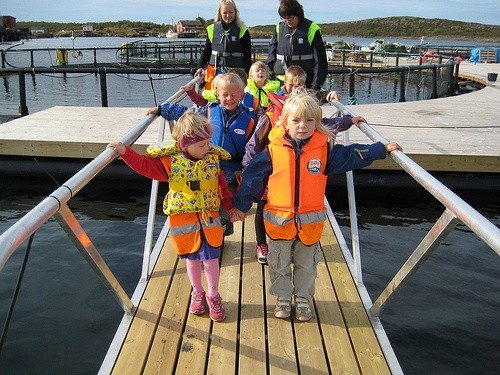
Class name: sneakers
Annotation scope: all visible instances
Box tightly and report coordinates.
[205,289,225,322]
[189,287,206,315]
[274,296,291,318]
[294,296,312,321]
[256,244,268,263]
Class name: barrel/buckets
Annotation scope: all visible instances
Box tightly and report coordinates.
[488,73,498,81]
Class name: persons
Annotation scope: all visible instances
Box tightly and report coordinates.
[242,89,368,265]
[179,69,263,118]
[230,93,403,321]
[266,0,328,93]
[146,73,257,234]
[266,65,340,114]
[105,112,247,322]
[194,0,251,78]
[244,60,280,111]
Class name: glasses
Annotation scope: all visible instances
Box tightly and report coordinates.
[280,15,295,20]
